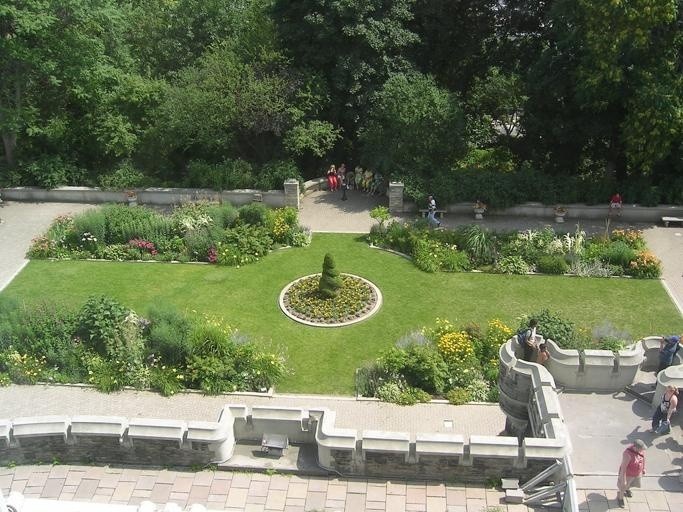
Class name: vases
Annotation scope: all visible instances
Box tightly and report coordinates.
[474,209,484,219]
[555,213,564,223]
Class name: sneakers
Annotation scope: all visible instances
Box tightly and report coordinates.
[617,490,633,508]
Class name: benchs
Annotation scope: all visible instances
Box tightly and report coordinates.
[419,208,446,218]
[662,215,683,226]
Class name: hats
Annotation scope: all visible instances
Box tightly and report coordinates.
[671,336,679,341]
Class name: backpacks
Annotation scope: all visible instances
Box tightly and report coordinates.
[518,329,532,347]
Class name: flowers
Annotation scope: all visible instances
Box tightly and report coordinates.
[473,200,487,209]
[553,205,569,214]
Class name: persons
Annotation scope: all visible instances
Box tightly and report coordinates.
[426,196,440,228]
[537,344,549,365]
[521,318,539,360]
[615,439,647,508]
[327,163,383,196]
[650,335,680,388]
[645,384,679,434]
[608,192,624,217]
